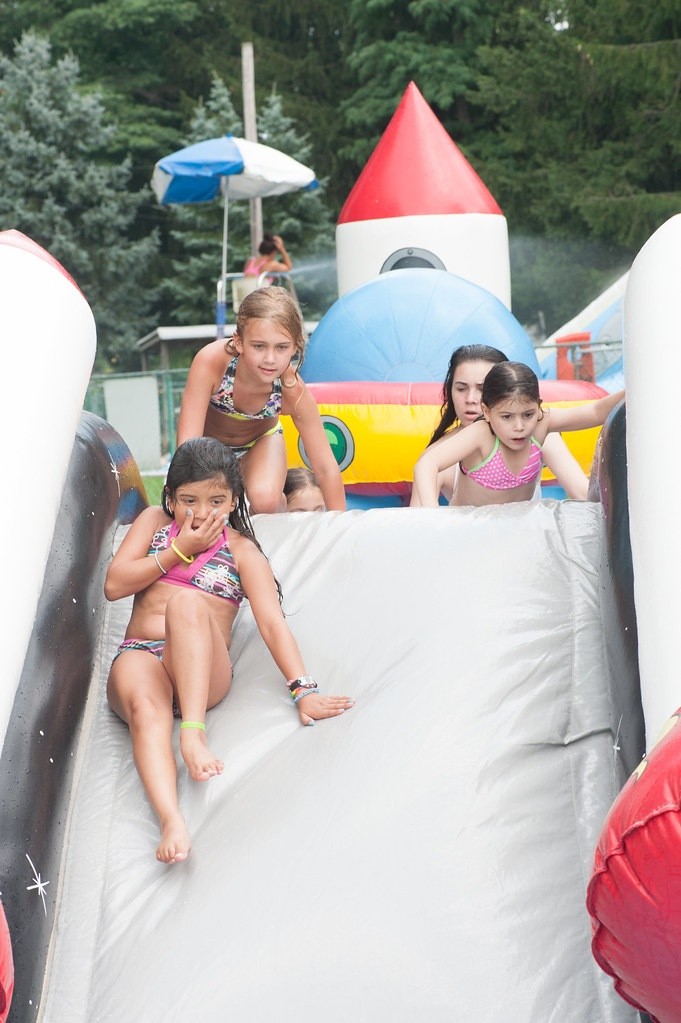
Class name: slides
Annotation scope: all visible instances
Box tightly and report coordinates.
[23,496,649,1023]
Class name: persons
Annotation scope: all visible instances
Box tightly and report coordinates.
[240,235,292,283]
[410,345,625,507]
[175,283,348,510]
[101,436,356,866]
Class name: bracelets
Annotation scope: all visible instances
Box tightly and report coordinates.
[171,539,195,563]
[153,549,167,575]
[286,675,320,704]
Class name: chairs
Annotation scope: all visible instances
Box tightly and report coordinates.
[218,271,302,342]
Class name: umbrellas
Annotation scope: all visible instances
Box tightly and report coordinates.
[150,131,321,341]
